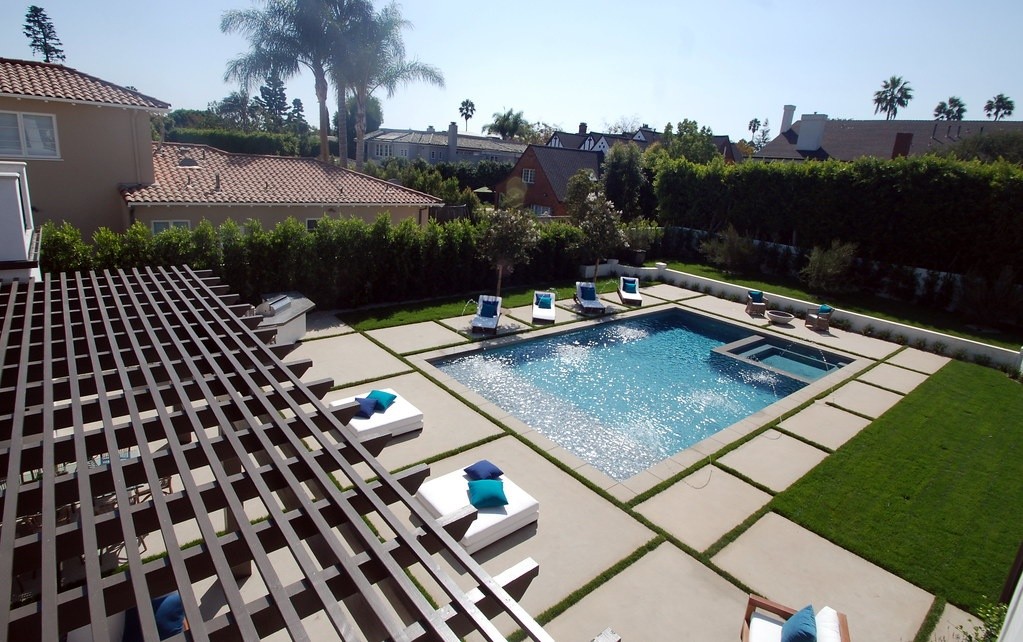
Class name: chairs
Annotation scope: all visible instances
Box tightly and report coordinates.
[471,295,502,335]
[532,291,556,325]
[740,593,850,642]
[617,276,642,307]
[805,307,835,332]
[745,294,768,317]
[573,281,605,314]
[23,447,176,567]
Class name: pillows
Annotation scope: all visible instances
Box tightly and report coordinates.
[481,303,495,318]
[750,291,766,303]
[468,480,509,508]
[623,278,636,290]
[483,301,499,316]
[463,459,504,480]
[122,590,186,642]
[580,286,595,298]
[366,390,396,411]
[583,289,596,300]
[538,297,551,308]
[818,305,831,318]
[625,283,636,293]
[67,611,126,642]
[781,604,817,642]
[535,293,550,305]
[354,397,376,419]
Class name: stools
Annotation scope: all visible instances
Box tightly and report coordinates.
[415,463,539,555]
[329,388,424,443]
